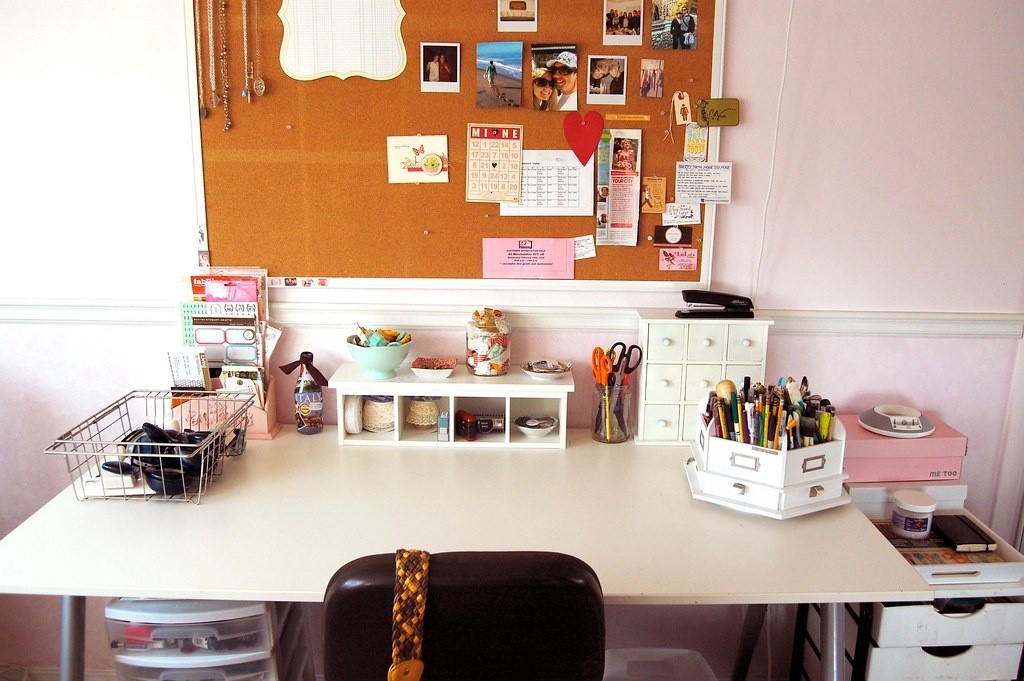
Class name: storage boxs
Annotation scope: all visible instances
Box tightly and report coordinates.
[835,409,970,521]
[170,374,283,439]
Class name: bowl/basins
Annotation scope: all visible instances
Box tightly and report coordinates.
[345,334,413,379]
[512,415,559,438]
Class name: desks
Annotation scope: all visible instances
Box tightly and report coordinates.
[0,421,934,681]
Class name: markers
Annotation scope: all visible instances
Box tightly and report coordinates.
[819,414,830,439]
[591,347,613,439]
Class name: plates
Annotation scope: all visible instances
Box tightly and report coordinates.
[409,357,457,380]
[519,360,573,381]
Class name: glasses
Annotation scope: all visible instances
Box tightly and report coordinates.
[533,77,554,89]
[551,66,574,76]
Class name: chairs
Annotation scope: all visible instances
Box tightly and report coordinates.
[320,552,606,681]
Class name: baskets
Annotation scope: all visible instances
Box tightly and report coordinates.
[43,390,256,505]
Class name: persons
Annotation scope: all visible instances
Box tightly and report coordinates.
[605,9,640,35]
[653,5,695,50]
[607,60,625,94]
[532,51,578,111]
[597,214,608,227]
[485,61,498,88]
[590,61,610,93]
[427,56,453,82]
[613,138,636,170]
[599,187,609,203]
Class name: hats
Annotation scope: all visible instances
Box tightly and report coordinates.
[676,12,682,14]
[546,51,578,68]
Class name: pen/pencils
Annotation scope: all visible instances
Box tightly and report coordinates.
[731,392,759,446]
[787,415,794,450]
[713,398,735,441]
[773,399,784,449]
[744,377,750,400]
[753,389,778,448]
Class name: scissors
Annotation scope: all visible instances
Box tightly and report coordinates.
[609,343,642,418]
[607,371,627,438]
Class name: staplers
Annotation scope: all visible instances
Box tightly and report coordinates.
[675,290,754,318]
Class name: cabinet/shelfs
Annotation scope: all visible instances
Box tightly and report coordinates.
[631,308,771,449]
[327,363,576,449]
[798,581,1024,681]
[104,597,316,681]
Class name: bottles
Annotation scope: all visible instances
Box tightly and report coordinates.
[589,381,633,444]
[293,352,324,435]
[890,488,937,539]
[466,315,511,376]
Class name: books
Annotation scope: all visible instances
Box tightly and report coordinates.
[928,514,998,553]
